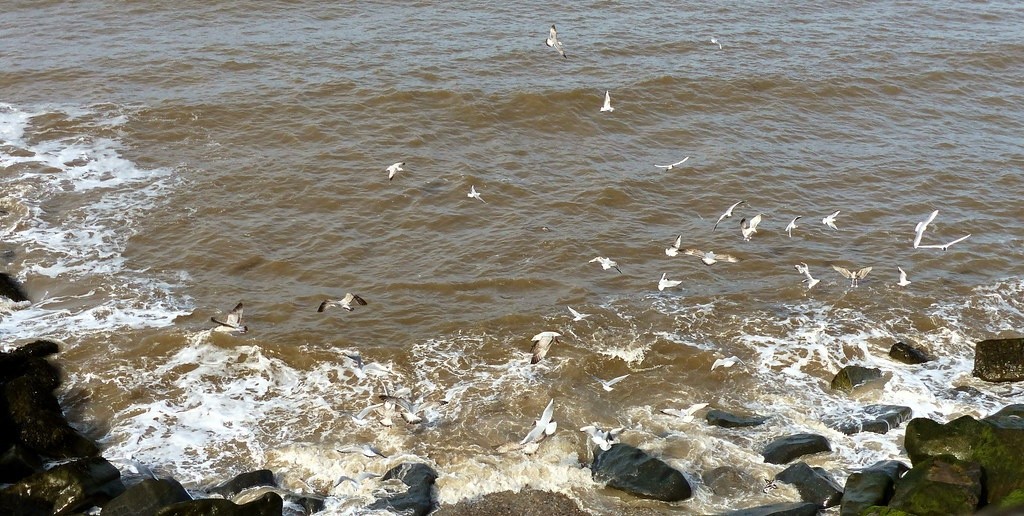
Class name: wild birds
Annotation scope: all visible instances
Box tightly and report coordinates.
[211,157,971,500]
[600,90,614,113]
[546,26,566,58]
